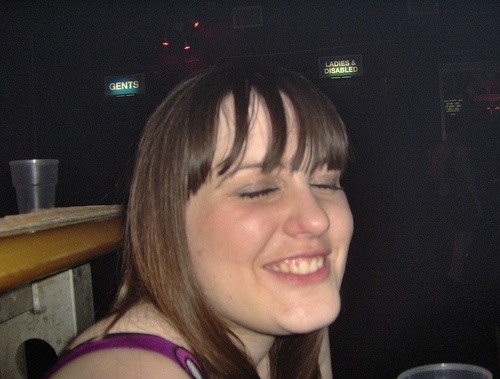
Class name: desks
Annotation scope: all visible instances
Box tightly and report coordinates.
[0,213,131,379]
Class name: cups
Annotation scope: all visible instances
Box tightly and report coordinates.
[9,158,59,219]
[397,363,492,379]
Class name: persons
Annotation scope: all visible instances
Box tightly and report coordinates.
[37,63,356,379]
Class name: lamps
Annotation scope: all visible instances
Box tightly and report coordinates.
[160,13,203,51]
